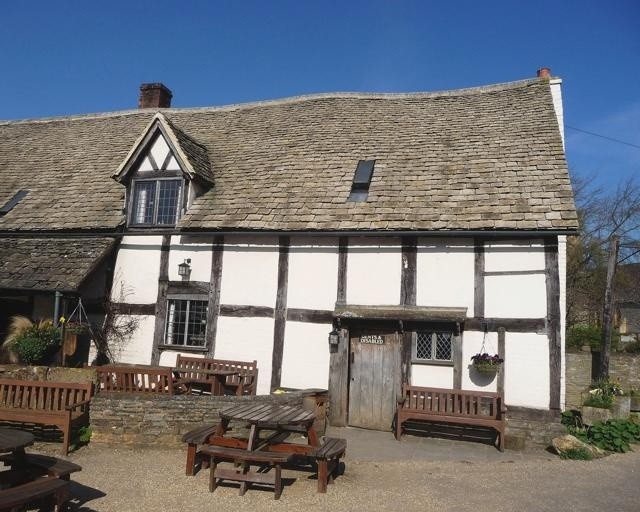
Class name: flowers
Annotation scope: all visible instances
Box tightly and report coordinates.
[585,375,625,409]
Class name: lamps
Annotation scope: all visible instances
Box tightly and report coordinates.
[178,259,191,276]
[329,318,340,353]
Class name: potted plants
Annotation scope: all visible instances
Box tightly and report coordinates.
[626,387,640,411]
[64,321,90,359]
[470,353,504,375]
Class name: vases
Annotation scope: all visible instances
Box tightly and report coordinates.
[582,395,631,427]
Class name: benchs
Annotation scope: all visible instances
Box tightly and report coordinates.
[246,424,309,438]
[310,436,346,493]
[168,354,258,396]
[396,382,506,452]
[181,424,235,476]
[0,477,70,512]
[83,362,172,398]
[200,444,292,500]
[0,378,93,456]
[0,453,81,481]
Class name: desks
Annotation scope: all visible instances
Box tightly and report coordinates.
[135,364,239,396]
[0,426,35,457]
[202,404,335,484]
